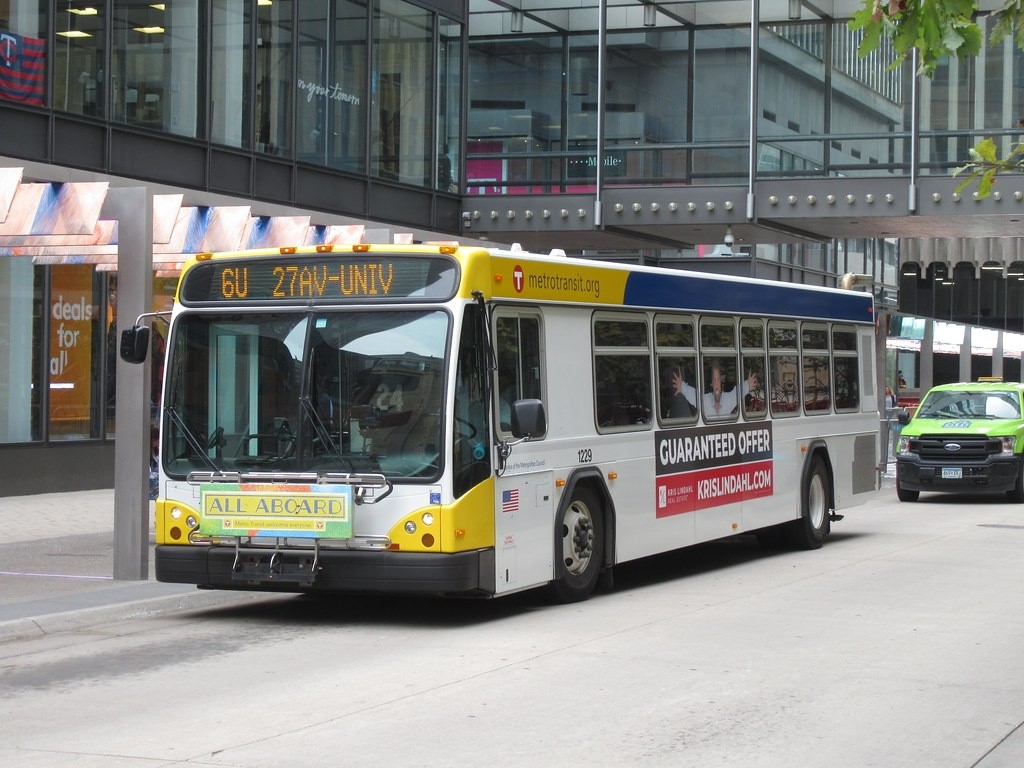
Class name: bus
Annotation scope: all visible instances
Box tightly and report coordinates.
[120,237,891,610]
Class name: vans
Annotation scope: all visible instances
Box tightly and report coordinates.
[893,374,1024,504]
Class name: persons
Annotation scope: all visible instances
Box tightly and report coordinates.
[671,363,758,416]
[441,366,513,463]
[885,386,897,429]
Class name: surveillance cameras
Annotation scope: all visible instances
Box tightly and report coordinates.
[724,234,734,247]
